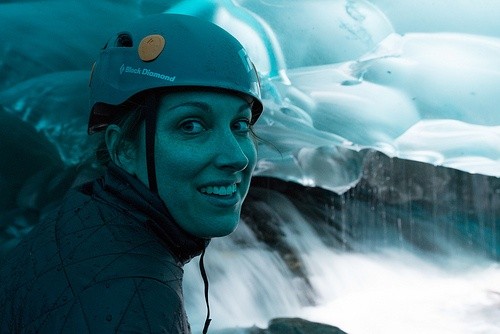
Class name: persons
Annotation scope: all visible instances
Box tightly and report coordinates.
[0,15,263,333]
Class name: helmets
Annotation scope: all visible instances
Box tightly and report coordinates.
[87,14,264,135]
